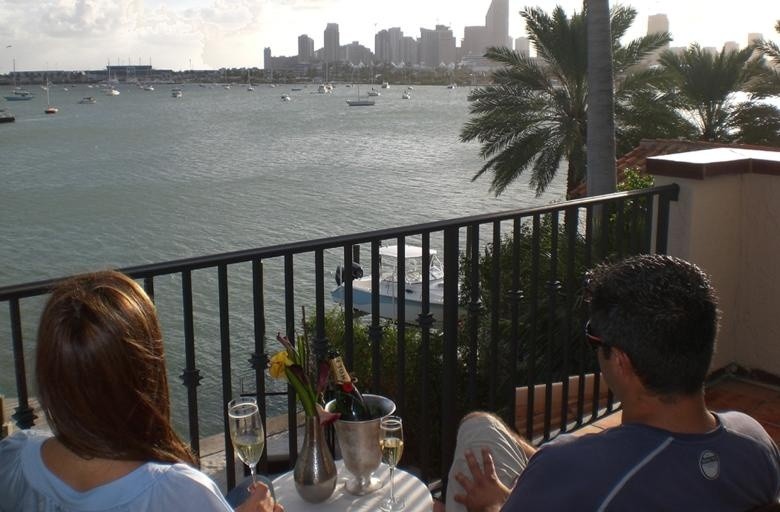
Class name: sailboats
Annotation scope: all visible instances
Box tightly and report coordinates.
[44,77,59,114]
[345,62,377,106]
[2,58,35,100]
[317,61,335,94]
[367,69,381,97]
[87,59,121,96]
[247,68,255,91]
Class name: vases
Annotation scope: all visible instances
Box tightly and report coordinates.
[293,414,337,503]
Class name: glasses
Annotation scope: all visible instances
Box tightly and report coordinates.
[585,320,601,344]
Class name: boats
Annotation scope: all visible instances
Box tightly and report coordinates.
[281,93,291,102]
[78,96,97,104]
[63,87,68,92]
[402,84,413,100]
[269,83,284,88]
[382,81,390,89]
[199,81,233,89]
[143,85,155,91]
[447,84,454,89]
[0,108,16,123]
[329,244,469,328]
[172,90,182,98]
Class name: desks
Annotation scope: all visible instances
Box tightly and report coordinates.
[268,460,434,512]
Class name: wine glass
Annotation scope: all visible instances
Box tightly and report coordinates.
[378,414,407,512]
[228,396,265,494]
[326,395,398,498]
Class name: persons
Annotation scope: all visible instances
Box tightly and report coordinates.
[440,254,780,511]
[1,271,284,510]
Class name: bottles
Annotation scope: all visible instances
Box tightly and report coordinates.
[327,350,368,419]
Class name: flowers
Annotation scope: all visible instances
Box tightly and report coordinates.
[269,305,343,424]
